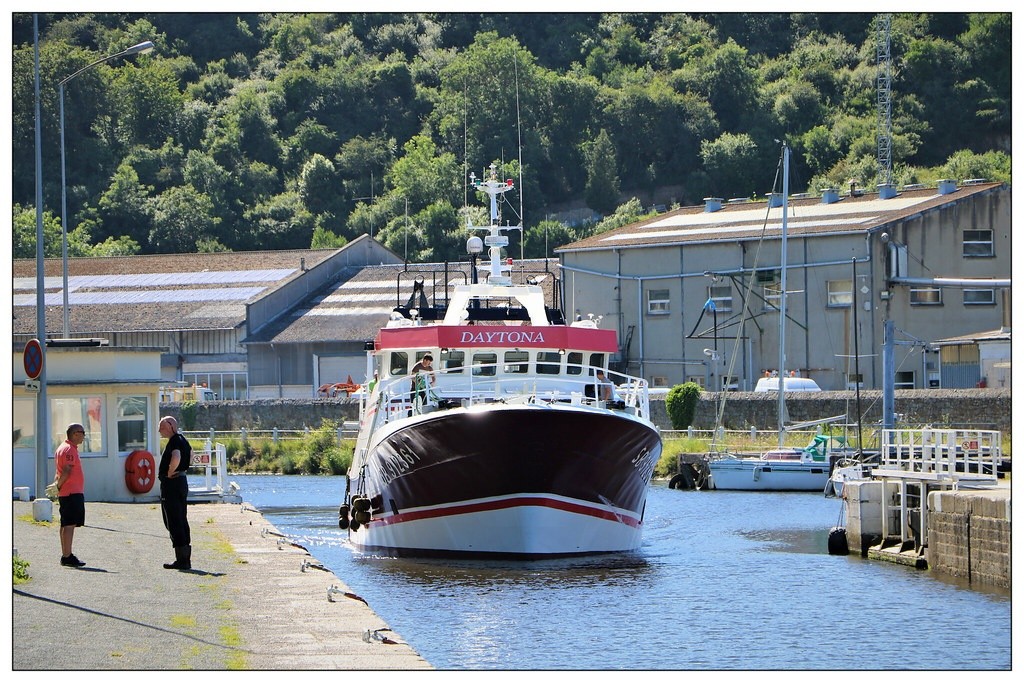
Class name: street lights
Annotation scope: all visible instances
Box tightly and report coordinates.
[57,38,158,338]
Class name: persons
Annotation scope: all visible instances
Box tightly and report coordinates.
[369,370,377,391]
[202,382,207,388]
[346,377,353,397]
[408,354,436,417]
[44,424,86,566]
[158,416,194,570]
[597,370,614,409]
[979,377,986,388]
[760,369,797,378]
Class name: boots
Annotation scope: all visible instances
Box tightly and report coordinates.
[163,546,192,569]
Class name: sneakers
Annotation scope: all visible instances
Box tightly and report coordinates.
[61,553,86,566]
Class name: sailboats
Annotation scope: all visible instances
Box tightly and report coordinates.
[703,138,835,493]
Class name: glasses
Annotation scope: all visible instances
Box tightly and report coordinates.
[73,431,84,435]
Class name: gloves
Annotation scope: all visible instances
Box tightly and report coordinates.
[45,481,60,498]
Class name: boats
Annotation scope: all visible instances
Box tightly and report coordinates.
[337,53,660,565]
